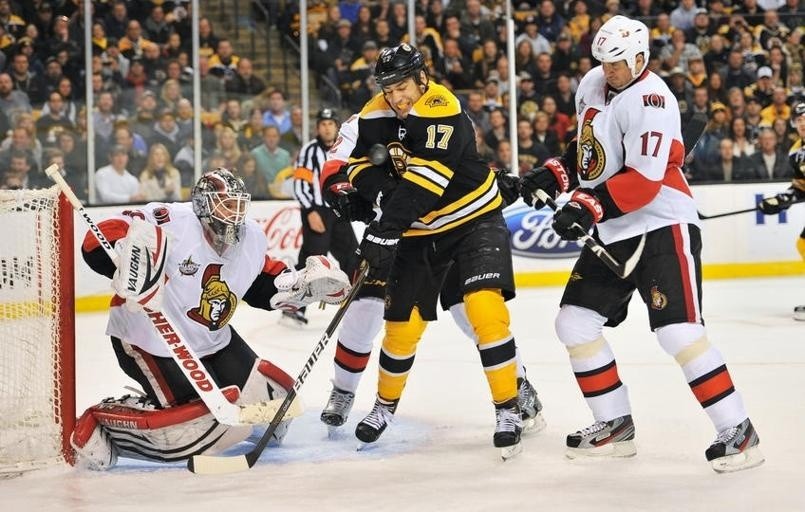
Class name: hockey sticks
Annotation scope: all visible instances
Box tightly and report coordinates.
[695,200,802,220]
[532,188,649,281]
[45,164,306,425]
[187,263,371,474]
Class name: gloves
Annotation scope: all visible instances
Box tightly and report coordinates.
[272,254,350,310]
[551,187,604,241]
[112,223,168,310]
[317,178,359,217]
[519,157,567,211]
[758,192,791,216]
[353,233,402,282]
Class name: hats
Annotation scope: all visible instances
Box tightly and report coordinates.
[757,66,772,80]
[317,106,341,124]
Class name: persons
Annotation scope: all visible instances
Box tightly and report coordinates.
[292,107,360,285]
[520,14,760,460]
[321,112,544,425]
[757,101,804,321]
[74,166,353,470]
[321,39,529,448]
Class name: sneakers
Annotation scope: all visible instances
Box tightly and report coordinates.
[355,395,400,443]
[320,384,356,426]
[491,379,542,449]
[705,417,761,460]
[567,413,636,448]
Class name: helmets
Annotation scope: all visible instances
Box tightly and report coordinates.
[791,102,805,124]
[374,44,431,89]
[590,12,652,76]
[192,164,250,246]
